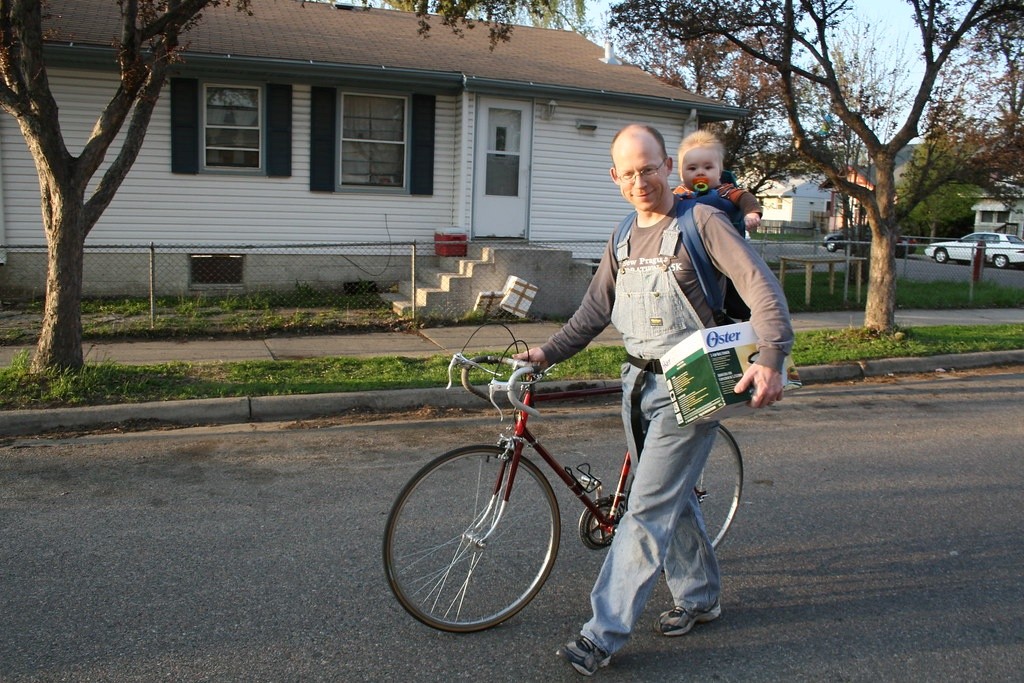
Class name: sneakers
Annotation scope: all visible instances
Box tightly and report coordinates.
[555,636,611,676]
[654,596,721,635]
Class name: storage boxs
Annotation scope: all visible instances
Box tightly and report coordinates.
[434,233,467,256]
[499,275,538,318]
[659,321,802,425]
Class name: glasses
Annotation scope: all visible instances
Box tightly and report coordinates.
[615,157,666,183]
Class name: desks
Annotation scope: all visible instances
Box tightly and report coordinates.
[778,252,867,306]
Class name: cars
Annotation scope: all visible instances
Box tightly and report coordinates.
[924,232,1024,269]
[823,225,917,258]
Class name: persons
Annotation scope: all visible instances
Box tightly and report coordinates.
[511,125,794,676]
[668,130,764,323]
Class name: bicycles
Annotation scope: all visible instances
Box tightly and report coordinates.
[382,323,743,633]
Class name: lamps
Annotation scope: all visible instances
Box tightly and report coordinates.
[541,100,557,121]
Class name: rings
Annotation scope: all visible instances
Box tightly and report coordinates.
[770,400,776,403]
